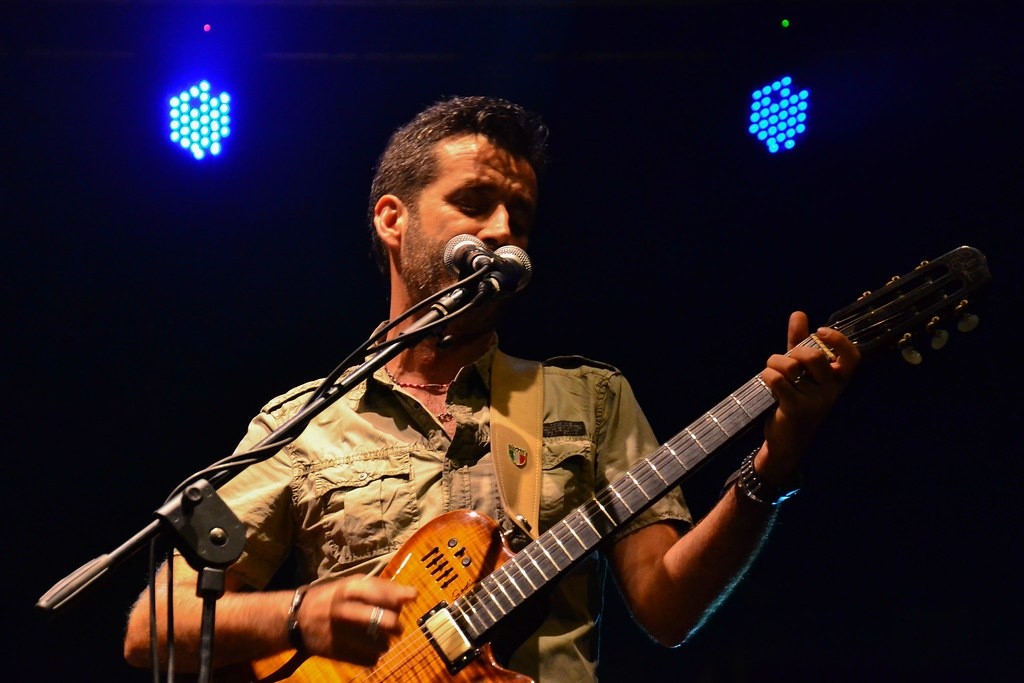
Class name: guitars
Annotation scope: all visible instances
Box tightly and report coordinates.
[244,240,985,683]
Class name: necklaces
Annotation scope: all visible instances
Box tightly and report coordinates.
[385,363,448,388]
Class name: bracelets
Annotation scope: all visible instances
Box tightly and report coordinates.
[724,447,804,505]
[288,584,313,656]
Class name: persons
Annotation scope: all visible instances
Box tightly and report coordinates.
[123,97,861,683]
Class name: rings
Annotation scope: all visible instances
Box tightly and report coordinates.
[792,369,809,387]
[367,606,383,636]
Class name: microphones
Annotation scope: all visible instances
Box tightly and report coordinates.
[479,245,532,296]
[443,234,492,275]
